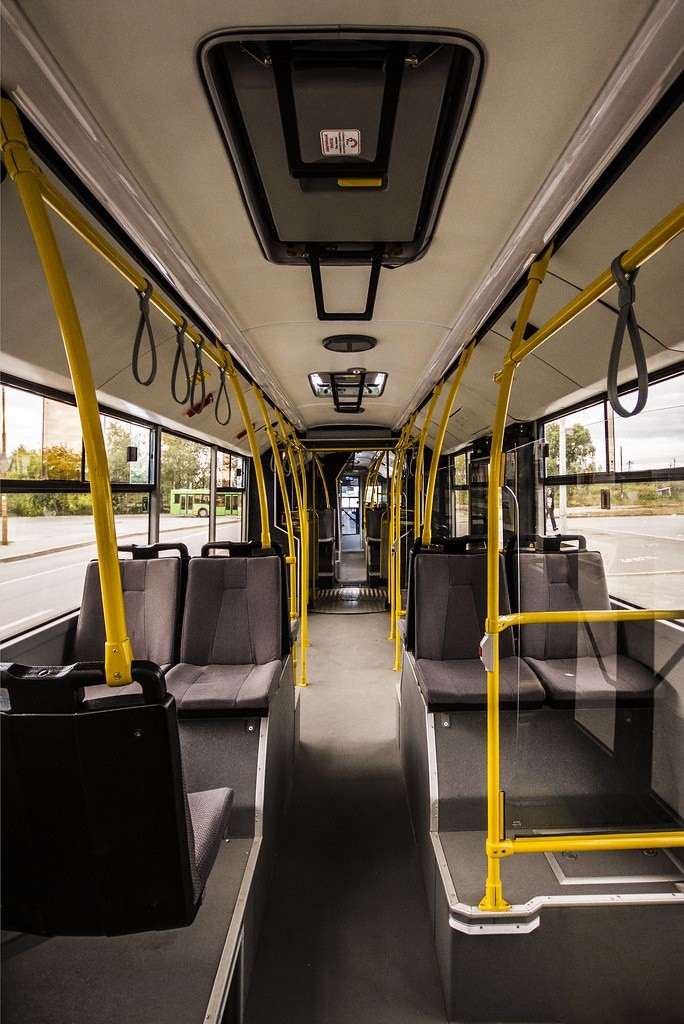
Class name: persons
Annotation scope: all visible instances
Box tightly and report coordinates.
[545,488,558,531]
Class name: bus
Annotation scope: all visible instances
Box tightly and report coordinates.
[169,486,242,517]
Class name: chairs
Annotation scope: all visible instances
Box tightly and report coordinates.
[393,534,657,703]
[0,537,299,968]
[312,507,387,582]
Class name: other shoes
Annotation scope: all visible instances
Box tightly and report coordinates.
[553,527,557,531]
